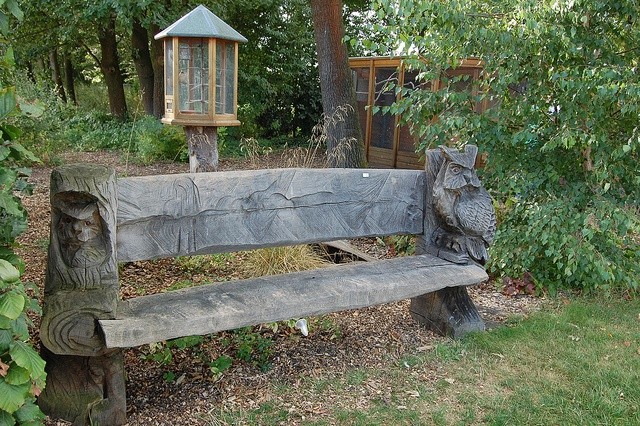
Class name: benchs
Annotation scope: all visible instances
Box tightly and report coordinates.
[33,144,497,426]
